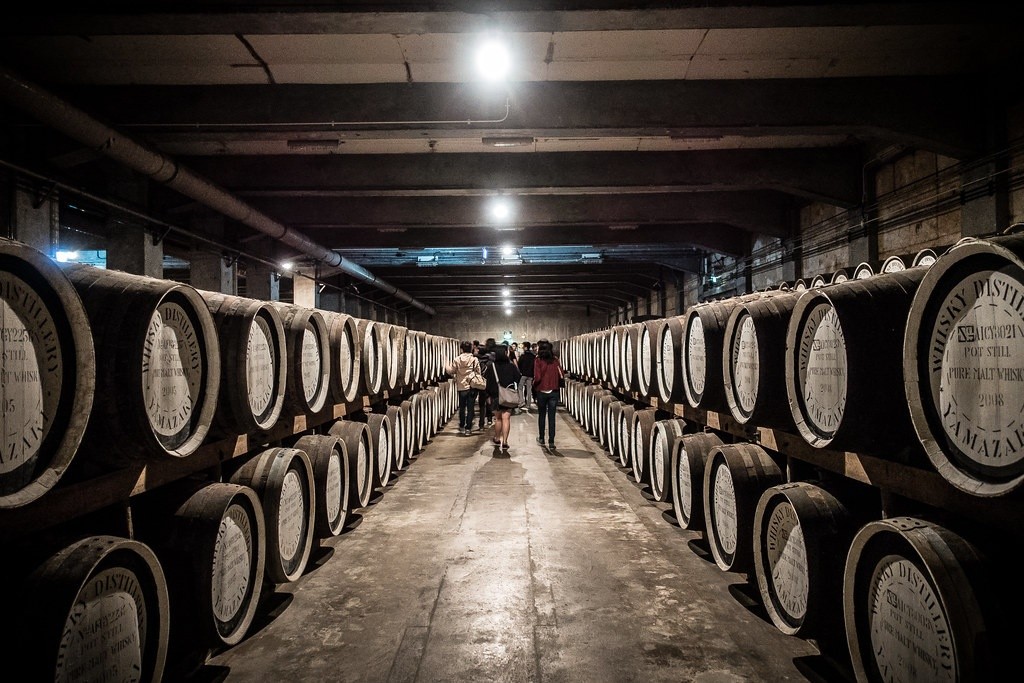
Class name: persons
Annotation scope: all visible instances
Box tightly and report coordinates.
[484,345,521,449]
[531,338,564,449]
[446,338,554,435]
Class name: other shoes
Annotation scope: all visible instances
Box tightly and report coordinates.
[460,428,465,433]
[514,407,522,414]
[502,444,508,450]
[488,422,492,425]
[525,405,530,408]
[465,429,473,436]
[549,444,556,450]
[518,402,526,408]
[493,437,501,446]
[536,436,545,446]
[479,427,484,431]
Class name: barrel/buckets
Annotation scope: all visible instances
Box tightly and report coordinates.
[0,235,461,683]
[556,218,1024,683]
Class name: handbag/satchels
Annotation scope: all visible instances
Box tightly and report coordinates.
[555,356,565,388]
[466,360,486,391]
[498,386,519,408]
